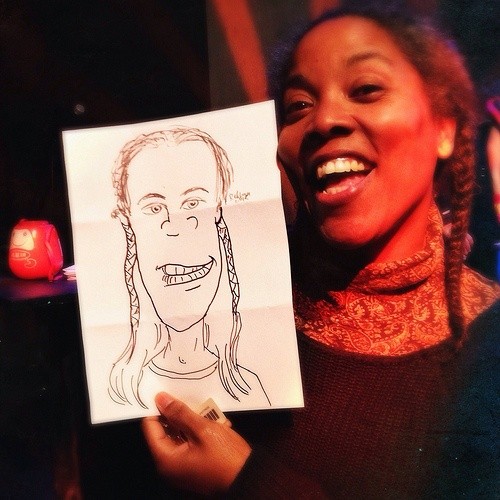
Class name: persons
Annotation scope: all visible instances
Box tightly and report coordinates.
[142,1,500,500]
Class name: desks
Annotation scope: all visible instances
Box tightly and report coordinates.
[0,279,77,310]
